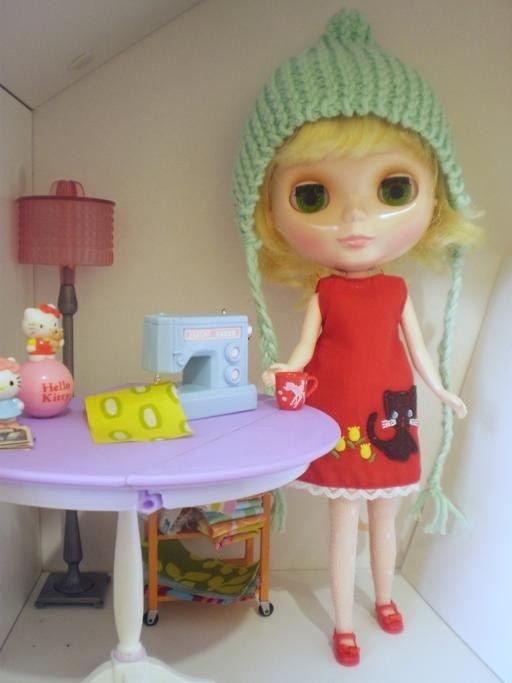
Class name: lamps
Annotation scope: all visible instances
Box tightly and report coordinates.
[15,179,115,605]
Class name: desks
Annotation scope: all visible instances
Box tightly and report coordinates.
[2,383,338,682]
